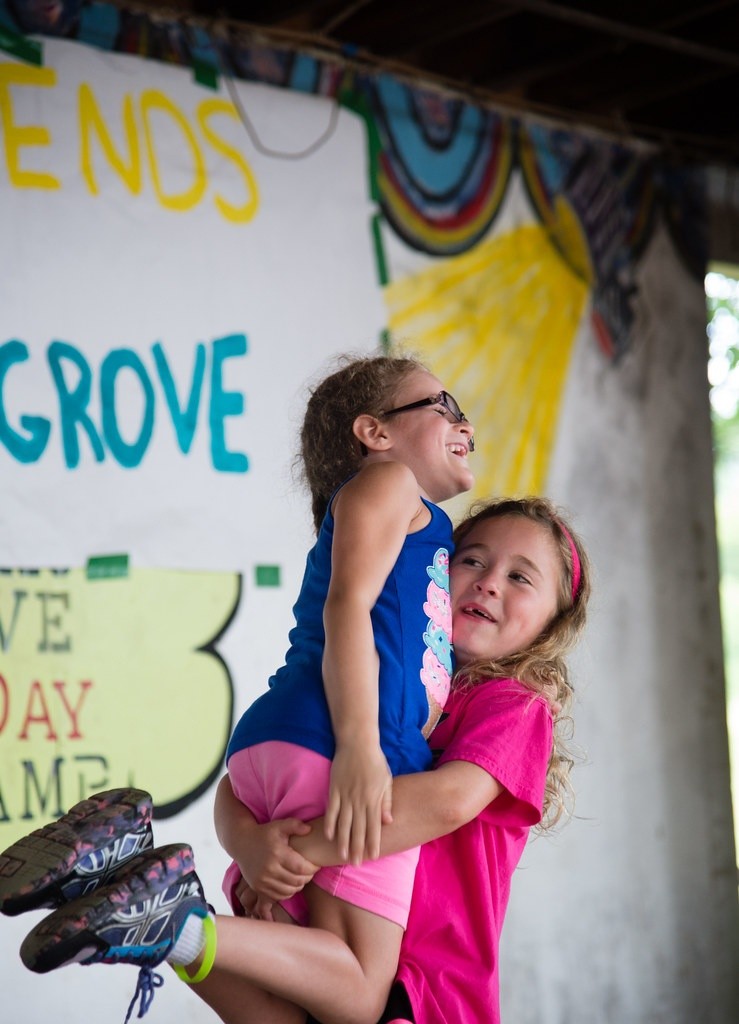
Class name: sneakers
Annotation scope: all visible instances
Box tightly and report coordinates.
[0,788,154,916]
[19,842,216,1024]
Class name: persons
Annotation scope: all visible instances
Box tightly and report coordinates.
[214,497,590,1024]
[0,357,475,1023]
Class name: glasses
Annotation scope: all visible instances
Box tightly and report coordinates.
[359,390,475,457]
